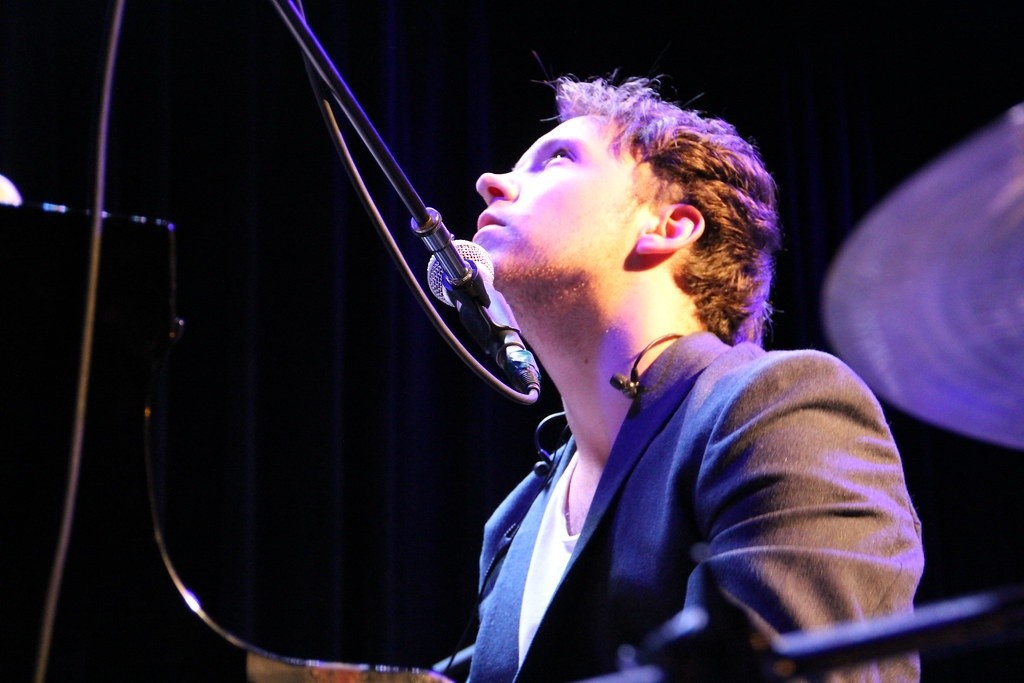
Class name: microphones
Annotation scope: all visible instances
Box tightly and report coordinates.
[426,240,541,393]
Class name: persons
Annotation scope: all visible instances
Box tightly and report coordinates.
[467,79,926,682]
